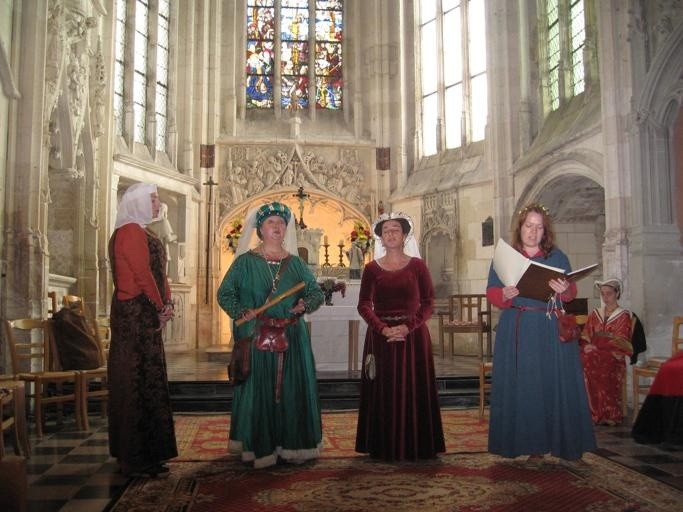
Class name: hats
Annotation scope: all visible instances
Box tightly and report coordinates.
[592,277,624,296]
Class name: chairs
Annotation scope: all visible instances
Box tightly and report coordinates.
[633,316,683,424]
[574,314,629,417]
[480,361,493,415]
[0,293,107,512]
[436,294,493,362]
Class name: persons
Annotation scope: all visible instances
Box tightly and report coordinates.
[348,211,452,464]
[103,180,179,479]
[579,276,645,429]
[213,201,327,471]
[484,202,598,471]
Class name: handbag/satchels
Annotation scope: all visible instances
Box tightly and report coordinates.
[253,325,289,354]
[223,337,253,386]
[363,326,377,382]
[554,305,583,345]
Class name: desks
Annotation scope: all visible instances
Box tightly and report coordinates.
[304,305,364,371]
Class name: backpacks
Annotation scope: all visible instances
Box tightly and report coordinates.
[49,305,105,373]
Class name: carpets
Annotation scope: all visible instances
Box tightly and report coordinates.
[103,409,683,512]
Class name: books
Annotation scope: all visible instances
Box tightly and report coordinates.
[514,258,598,303]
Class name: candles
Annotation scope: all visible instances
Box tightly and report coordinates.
[338,239,343,246]
[324,235,328,246]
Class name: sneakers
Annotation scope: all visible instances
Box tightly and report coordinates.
[128,464,170,478]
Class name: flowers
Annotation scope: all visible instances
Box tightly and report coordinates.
[352,220,372,254]
[221,219,241,254]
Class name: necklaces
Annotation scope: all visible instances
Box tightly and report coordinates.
[258,242,286,294]
